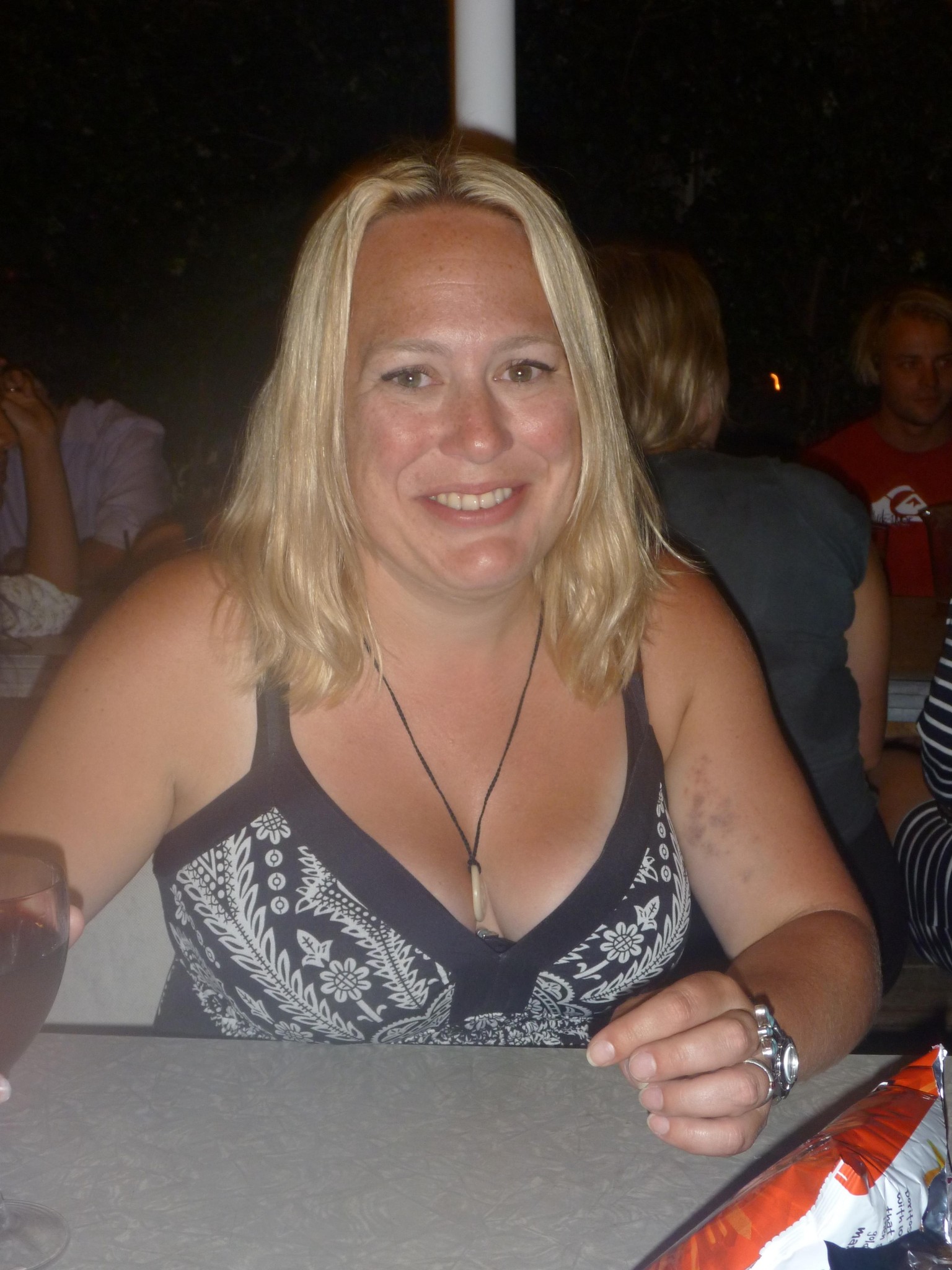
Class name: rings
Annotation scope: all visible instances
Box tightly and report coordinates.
[754,1004,778,1059]
[743,1056,772,1104]
[7,387,21,392]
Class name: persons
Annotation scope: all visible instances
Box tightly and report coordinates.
[580,234,890,999]
[0,129,882,1157]
[800,287,952,597]
[0,334,175,637]
[892,597,952,969]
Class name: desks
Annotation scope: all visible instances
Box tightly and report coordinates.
[0,1031,952,1270]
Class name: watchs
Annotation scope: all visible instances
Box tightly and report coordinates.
[772,1016,799,1101]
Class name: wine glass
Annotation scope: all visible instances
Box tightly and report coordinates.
[0,849,71,1269]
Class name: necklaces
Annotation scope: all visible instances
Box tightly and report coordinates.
[362,601,545,921]
